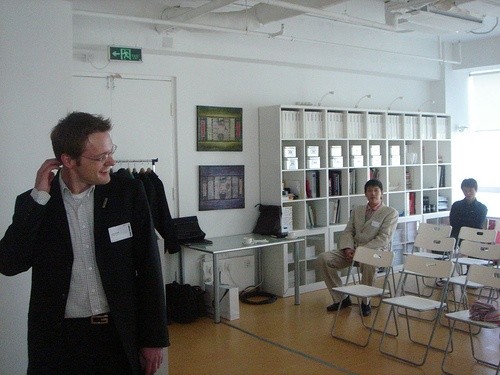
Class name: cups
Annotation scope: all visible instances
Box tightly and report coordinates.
[242,237,252,245]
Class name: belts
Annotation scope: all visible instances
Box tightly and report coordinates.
[62,312,114,325]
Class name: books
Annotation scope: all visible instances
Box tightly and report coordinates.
[370,168,380,180]
[423,196,436,213]
[329,171,341,196]
[438,193,448,211]
[438,165,445,187]
[329,199,341,225]
[406,192,415,215]
[349,169,356,194]
[306,201,316,229]
[306,171,320,198]
[406,170,412,190]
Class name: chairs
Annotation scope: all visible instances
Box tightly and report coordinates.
[330,218,500,375]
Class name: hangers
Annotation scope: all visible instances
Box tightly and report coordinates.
[127,160,152,179]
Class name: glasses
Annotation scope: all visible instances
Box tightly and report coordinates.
[70,144,118,161]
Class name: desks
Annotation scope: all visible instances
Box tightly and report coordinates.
[179,232,304,324]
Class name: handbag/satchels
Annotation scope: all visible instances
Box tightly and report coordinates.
[174,216,206,245]
[252,202,280,235]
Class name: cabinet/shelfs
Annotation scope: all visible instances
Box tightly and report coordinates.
[258,104,454,298]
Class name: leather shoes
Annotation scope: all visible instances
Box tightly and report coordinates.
[326,295,352,311]
[360,302,371,316]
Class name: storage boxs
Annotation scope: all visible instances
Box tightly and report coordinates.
[306,269,316,284]
[284,206,293,231]
[305,146,320,169]
[289,271,295,281]
[282,146,298,170]
[369,145,382,166]
[306,245,315,258]
[288,252,293,261]
[349,145,364,167]
[328,146,343,168]
[389,145,401,165]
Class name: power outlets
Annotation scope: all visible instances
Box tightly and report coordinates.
[206,261,231,274]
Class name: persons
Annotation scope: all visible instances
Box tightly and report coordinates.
[318,179,399,316]
[0,110,171,375]
[432,179,488,261]
